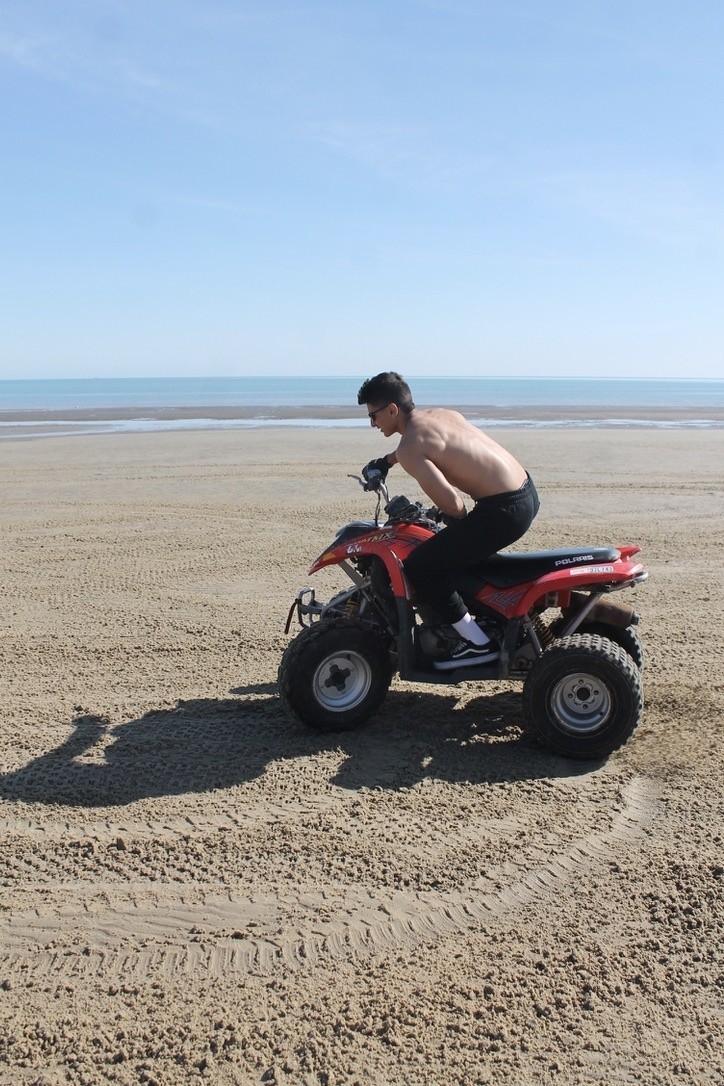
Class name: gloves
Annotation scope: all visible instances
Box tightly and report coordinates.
[362,456,392,491]
[442,506,467,525]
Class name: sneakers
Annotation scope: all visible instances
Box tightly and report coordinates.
[433,638,501,670]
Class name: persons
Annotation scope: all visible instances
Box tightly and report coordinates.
[358,372,540,669]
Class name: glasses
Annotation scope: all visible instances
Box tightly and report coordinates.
[368,406,388,420]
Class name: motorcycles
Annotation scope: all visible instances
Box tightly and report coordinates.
[278,468,649,759]
